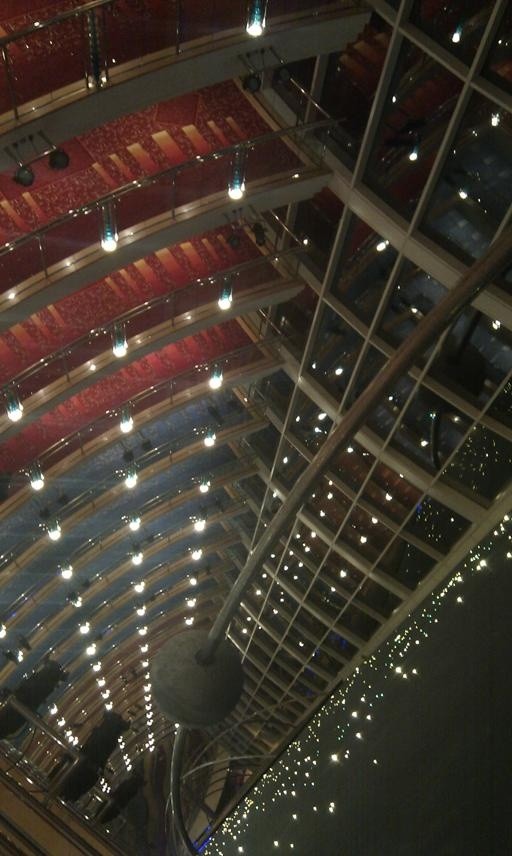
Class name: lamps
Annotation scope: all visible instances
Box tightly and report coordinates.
[3,0,288,819]
